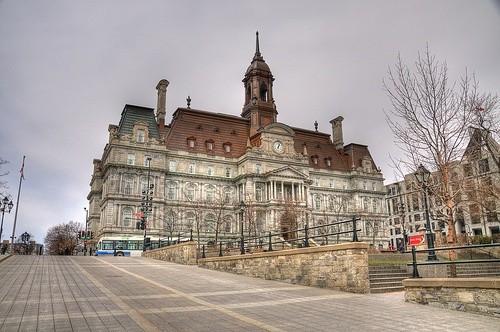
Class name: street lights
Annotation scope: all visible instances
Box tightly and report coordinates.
[239,200,247,254]
[84,207,88,231]
[141,157,154,252]
[413,162,440,261]
[0,196,14,239]
[95,236,191,256]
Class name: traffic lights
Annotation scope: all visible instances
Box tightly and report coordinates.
[91,231,94,239]
[82,231,85,240]
[87,231,91,240]
[78,230,81,239]
